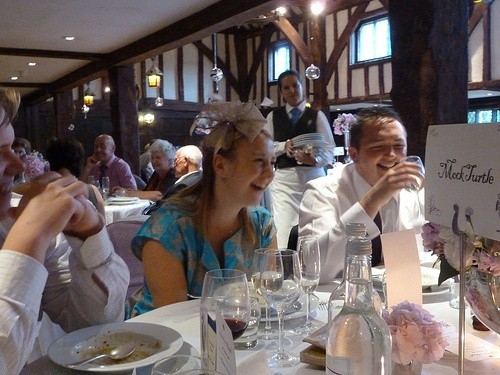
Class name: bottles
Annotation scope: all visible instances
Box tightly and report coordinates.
[328,222,383,323]
[325,237,393,375]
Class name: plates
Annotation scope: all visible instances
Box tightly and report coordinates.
[290,133,330,148]
[47,322,183,372]
[214,282,319,323]
[109,197,141,205]
[374,285,452,296]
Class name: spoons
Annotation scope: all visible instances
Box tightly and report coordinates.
[66,342,137,368]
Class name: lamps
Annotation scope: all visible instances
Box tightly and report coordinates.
[145,57,164,87]
[83,82,96,106]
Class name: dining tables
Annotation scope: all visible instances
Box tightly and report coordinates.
[19,260,500,375]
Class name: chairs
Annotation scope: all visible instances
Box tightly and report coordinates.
[104,221,146,302]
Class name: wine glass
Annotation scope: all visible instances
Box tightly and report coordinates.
[259,248,302,368]
[250,248,285,340]
[102,177,109,206]
[398,155,425,219]
[290,236,321,335]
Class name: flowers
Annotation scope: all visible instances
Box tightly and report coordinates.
[332,112,358,135]
[190,109,223,136]
[24,150,46,177]
[380,300,449,365]
[420,223,500,289]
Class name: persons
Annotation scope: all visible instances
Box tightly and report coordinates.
[262,69,336,273]
[296,104,432,285]
[0,85,132,375]
[9,134,204,258]
[132,106,277,340]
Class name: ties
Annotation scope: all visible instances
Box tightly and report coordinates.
[370,213,383,267]
[290,107,302,124]
[99,165,107,187]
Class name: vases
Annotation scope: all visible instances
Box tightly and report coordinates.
[391,358,422,375]
[342,131,352,164]
[454,265,500,336]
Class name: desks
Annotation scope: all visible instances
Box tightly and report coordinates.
[8,193,155,226]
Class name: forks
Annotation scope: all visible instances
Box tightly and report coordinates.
[313,291,329,305]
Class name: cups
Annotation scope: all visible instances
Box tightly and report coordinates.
[449,277,470,309]
[87,175,100,190]
[151,354,223,375]
[234,297,262,351]
[200,268,251,342]
[115,188,126,197]
